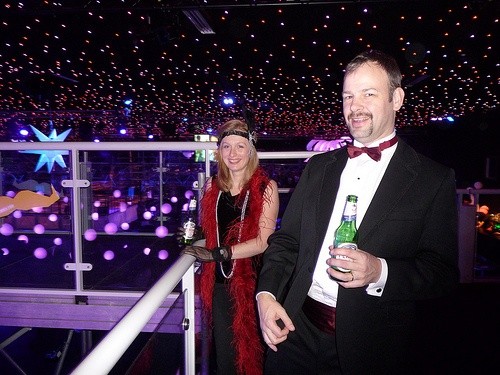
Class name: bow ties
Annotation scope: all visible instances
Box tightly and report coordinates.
[347,135,398,163]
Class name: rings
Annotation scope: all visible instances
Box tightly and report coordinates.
[349,271,354,281]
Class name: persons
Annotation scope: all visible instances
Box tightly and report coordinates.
[181,120,280,375]
[254,53,458,375]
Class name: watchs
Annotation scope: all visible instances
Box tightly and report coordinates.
[218,248,224,260]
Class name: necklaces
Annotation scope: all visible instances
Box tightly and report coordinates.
[216,190,250,279]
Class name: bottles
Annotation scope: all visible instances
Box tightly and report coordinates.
[330,195,359,282]
[179,196,198,246]
